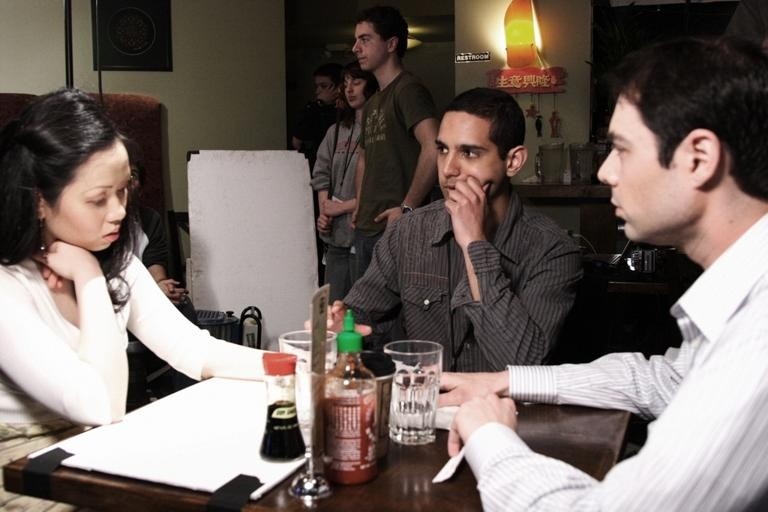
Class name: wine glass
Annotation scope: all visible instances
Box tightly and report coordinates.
[277,329,338,500]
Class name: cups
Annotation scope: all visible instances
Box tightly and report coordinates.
[534,139,566,183]
[381,338,444,448]
[569,142,594,185]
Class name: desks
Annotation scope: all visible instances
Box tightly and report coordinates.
[2,352,632,511]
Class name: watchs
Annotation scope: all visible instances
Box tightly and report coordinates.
[399,202,412,216]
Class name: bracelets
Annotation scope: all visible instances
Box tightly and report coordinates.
[155,277,168,285]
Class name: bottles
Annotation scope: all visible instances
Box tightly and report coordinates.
[259,352,307,463]
[319,306,382,485]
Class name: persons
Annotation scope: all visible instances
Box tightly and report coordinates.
[291,60,348,286]
[304,87,586,375]
[126,157,199,413]
[307,58,380,309]
[1,88,281,512]
[395,25,766,510]
[349,5,443,279]
